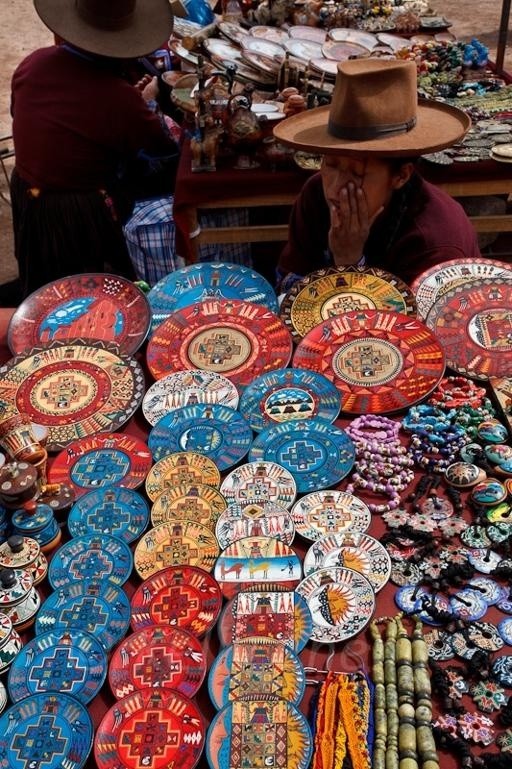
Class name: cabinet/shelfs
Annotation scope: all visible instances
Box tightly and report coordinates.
[0,308,512,769]
[172,28,512,266]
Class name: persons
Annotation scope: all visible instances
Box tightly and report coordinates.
[10,0,180,289]
[272,57,482,306]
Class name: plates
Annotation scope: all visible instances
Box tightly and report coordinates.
[490,143,512,157]
[190,66,218,99]
[390,38,410,52]
[249,25,291,47]
[409,257,512,324]
[327,28,379,54]
[94,685,207,769]
[0,691,95,769]
[371,42,394,55]
[240,48,280,78]
[48,533,133,591]
[248,419,356,494]
[434,32,456,43]
[215,498,295,548]
[238,368,343,435]
[292,309,447,414]
[212,535,305,609]
[290,490,372,542]
[375,32,409,45]
[216,20,253,44]
[108,622,208,703]
[240,35,287,59]
[144,452,221,504]
[151,482,228,536]
[249,103,279,112]
[202,38,259,71]
[68,485,150,545]
[48,432,152,503]
[302,528,391,595]
[9,626,109,709]
[308,58,342,82]
[130,563,223,642]
[295,566,377,644]
[423,278,512,384]
[6,272,153,358]
[220,582,314,656]
[146,260,280,342]
[211,55,277,91]
[219,461,296,510]
[284,38,327,62]
[145,298,293,396]
[134,520,220,581]
[409,35,434,45]
[168,38,199,64]
[35,577,132,653]
[321,40,370,59]
[204,692,315,767]
[288,25,329,46]
[277,263,419,348]
[0,337,146,453]
[209,635,307,712]
[147,403,254,471]
[255,112,287,120]
[142,368,239,428]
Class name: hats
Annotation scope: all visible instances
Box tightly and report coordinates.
[32,0,174,58]
[272,60,472,158]
